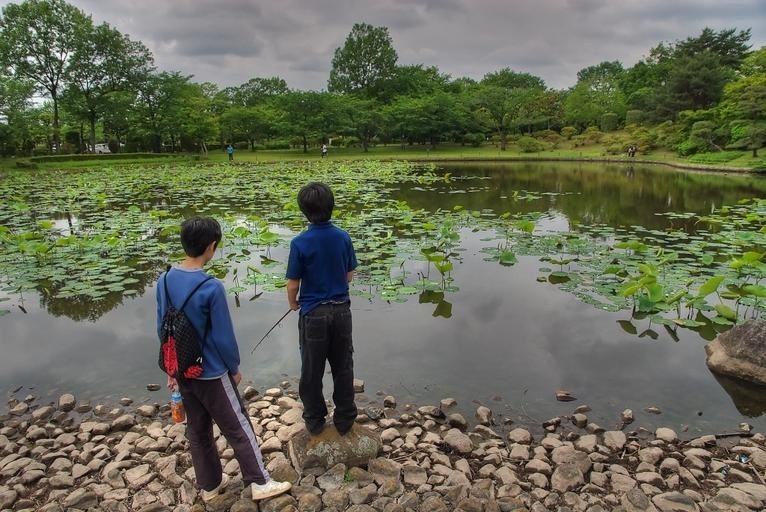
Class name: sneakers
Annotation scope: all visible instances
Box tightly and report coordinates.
[201,472,229,502]
[251,479,292,501]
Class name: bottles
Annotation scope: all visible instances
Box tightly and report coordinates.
[171,385,185,423]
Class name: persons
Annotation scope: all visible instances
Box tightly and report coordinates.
[287,181,360,437]
[321,144,327,158]
[632,146,637,157]
[157,216,292,502]
[227,145,234,162]
[628,147,632,156]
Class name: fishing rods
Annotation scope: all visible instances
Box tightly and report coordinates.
[250,300,300,356]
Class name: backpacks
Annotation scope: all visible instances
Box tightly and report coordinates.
[158,264,213,381]
